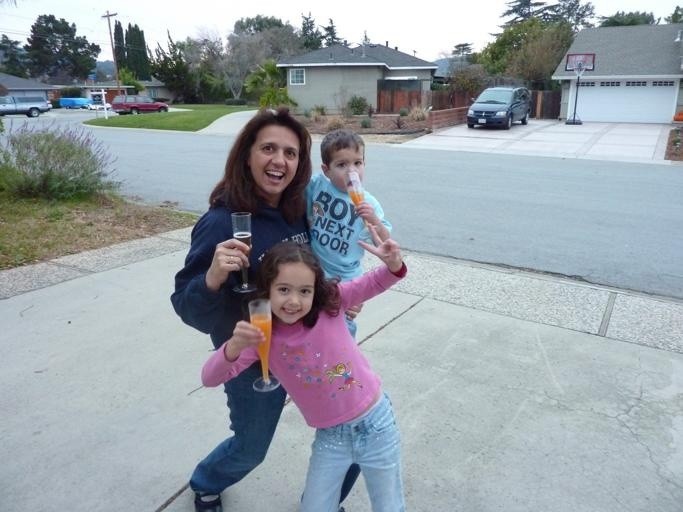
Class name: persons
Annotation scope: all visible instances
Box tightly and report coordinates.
[170,104,361,511]
[201,226,407,512]
[301,129,391,339]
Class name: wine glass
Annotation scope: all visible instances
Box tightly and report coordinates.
[248,298,279,393]
[231,212,257,295]
[341,170,379,242]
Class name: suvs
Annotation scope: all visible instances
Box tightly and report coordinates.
[111,95,169,115]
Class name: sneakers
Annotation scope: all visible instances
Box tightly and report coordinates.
[194,491,223,512]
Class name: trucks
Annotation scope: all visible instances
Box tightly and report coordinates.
[58,97,93,109]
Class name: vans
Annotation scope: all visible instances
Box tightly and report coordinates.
[0,95,52,117]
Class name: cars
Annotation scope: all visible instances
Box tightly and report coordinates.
[465,87,532,129]
[86,101,110,110]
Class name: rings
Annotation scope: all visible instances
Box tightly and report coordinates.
[229,256,234,265]
